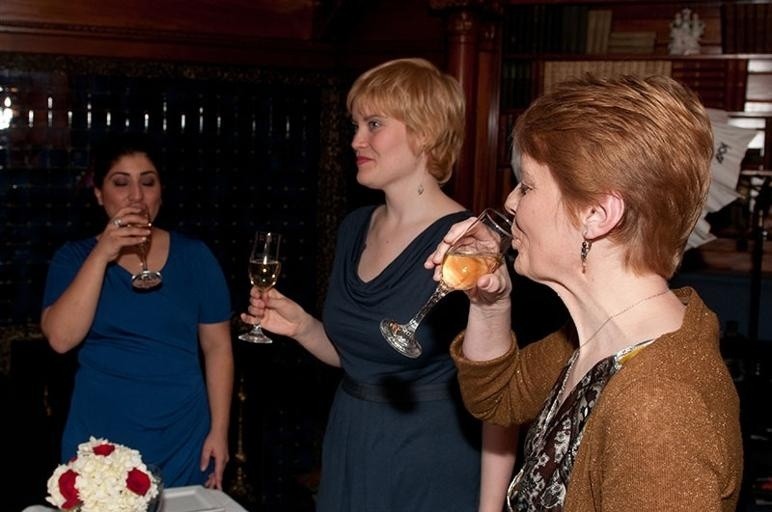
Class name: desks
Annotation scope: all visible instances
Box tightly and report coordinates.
[22,484,248,512]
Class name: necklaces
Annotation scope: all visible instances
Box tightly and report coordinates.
[570,287,671,364]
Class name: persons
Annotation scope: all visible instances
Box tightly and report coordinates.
[425,72,744,512]
[40,134,235,491]
[240,57,517,511]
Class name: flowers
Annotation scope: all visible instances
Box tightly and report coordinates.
[45,436,159,512]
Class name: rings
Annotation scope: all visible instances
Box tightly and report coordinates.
[113,217,122,228]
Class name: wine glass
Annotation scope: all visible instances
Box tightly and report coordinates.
[378,207,517,357]
[237,226,283,347]
[120,202,164,290]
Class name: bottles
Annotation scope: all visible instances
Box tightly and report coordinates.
[0,81,318,173]
